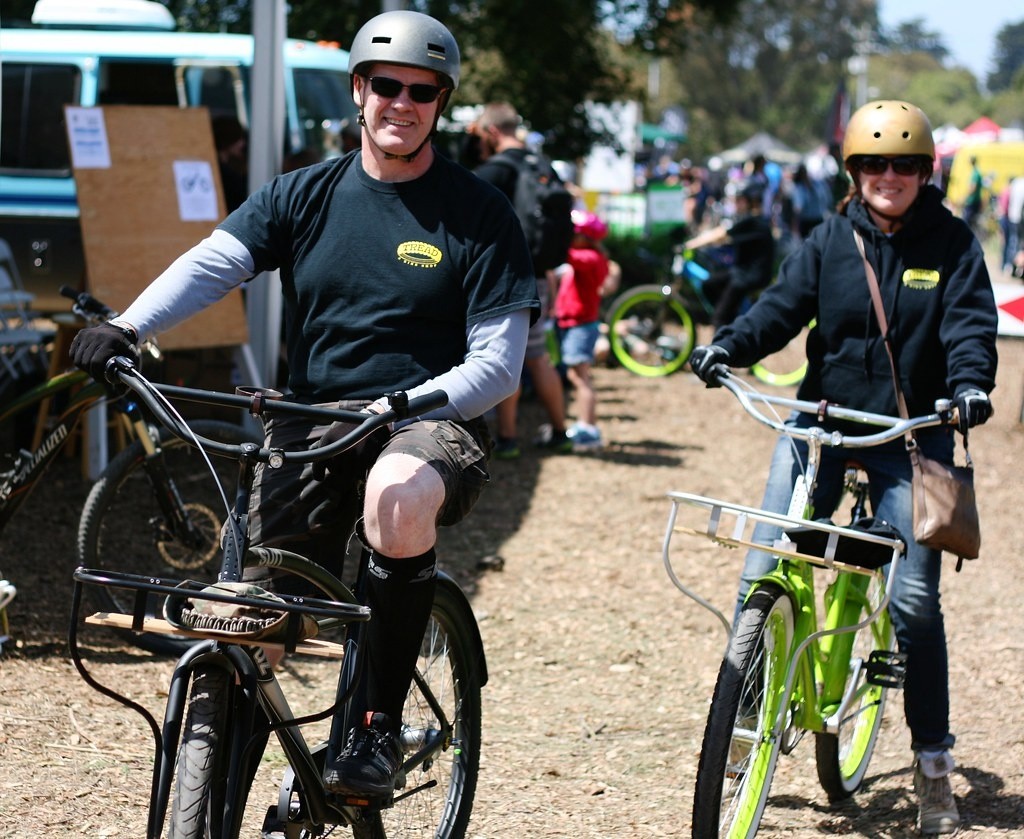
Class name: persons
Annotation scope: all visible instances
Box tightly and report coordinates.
[688,100,998,829]
[69,10,541,797]
[210,115,248,213]
[551,210,608,451]
[475,100,573,459]
[653,152,1024,339]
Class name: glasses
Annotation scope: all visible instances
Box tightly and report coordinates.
[364,75,443,104]
[854,154,925,175]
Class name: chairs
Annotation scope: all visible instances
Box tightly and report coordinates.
[0,237,57,380]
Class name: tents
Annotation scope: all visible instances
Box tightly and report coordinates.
[717,127,802,162]
[931,115,1024,206]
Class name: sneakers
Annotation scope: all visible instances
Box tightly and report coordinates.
[913,751,959,834]
[694,712,759,779]
[322,710,405,793]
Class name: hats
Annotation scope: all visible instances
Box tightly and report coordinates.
[162,579,319,643]
[572,209,608,242]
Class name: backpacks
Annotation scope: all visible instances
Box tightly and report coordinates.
[484,153,573,275]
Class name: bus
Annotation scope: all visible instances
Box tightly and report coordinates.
[0,0,363,297]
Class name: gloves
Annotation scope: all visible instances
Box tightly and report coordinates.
[313,409,392,482]
[688,342,728,390]
[950,388,991,432]
[68,321,140,388]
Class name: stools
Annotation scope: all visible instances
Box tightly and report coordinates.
[30,315,130,489]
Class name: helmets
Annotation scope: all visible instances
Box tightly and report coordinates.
[723,178,763,208]
[348,9,459,89]
[843,99,935,166]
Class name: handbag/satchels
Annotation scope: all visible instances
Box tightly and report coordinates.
[911,453,981,560]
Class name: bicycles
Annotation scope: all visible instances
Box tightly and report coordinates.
[606,246,817,388]
[70,353,488,839]
[663,363,952,839]
[0,285,261,658]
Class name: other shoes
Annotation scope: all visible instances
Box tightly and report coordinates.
[567,423,599,444]
[545,439,574,452]
[493,444,519,459]
